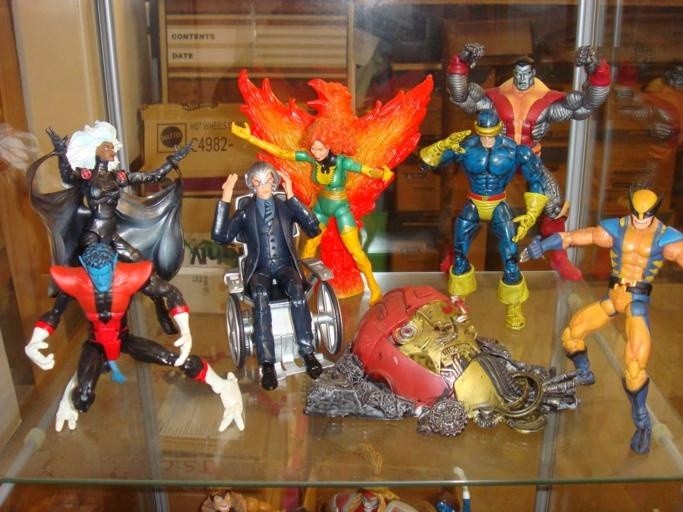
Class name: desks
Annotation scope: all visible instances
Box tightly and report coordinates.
[7,252,683,510]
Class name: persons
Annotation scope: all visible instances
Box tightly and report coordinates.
[231,120,395,304]
[20,120,242,436]
[20,36,682,511]
[214,163,322,389]
[419,42,612,332]
[519,189,681,453]
